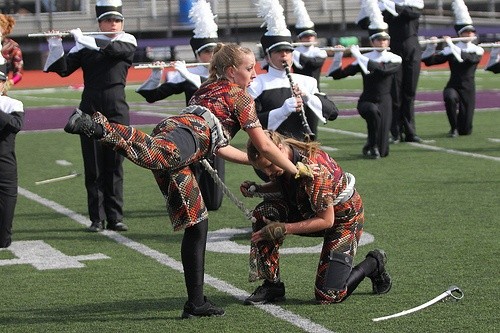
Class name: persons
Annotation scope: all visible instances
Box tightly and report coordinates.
[330,0,403,160]
[0,56,24,249]
[42,0,139,232]
[240,129,392,307]
[261,1,328,94]
[63,42,320,317]
[0,13,24,98]
[485,41,500,74]
[137,0,225,211]
[245,0,339,180]
[421,0,483,137]
[355,0,425,144]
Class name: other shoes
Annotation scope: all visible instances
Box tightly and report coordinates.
[449,129,458,137]
[89,220,105,232]
[404,135,422,142]
[362,148,368,157]
[106,222,128,231]
[64,107,96,138]
[392,136,402,143]
[370,144,380,159]
[182,296,226,319]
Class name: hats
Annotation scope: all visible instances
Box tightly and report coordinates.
[0,37,7,80]
[364,0,391,40]
[292,0,318,38]
[95,0,124,22]
[187,0,221,54]
[254,0,295,54]
[450,0,476,34]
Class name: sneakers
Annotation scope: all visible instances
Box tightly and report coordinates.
[245,282,285,305]
[366,248,392,294]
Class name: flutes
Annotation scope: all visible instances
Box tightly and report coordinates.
[320,46,390,51]
[418,37,477,44]
[134,63,211,70]
[255,41,318,47]
[281,60,316,142]
[28,30,126,38]
[478,43,500,48]
